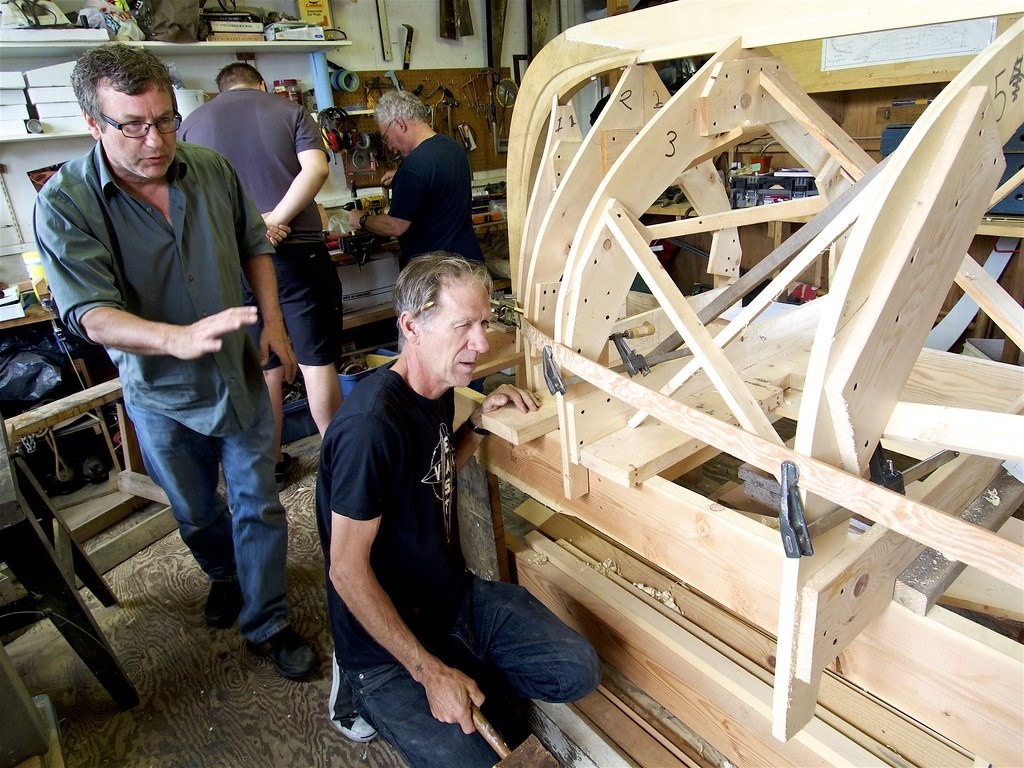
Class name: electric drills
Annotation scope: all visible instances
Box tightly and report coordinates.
[343,195,387,216]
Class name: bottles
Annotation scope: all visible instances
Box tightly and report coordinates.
[272,79,303,108]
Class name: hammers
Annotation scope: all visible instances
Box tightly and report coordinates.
[442,99,459,138]
[470,702,560,768]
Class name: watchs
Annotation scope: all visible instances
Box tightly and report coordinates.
[467,416,492,437]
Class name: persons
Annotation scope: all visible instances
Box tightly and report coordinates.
[32,41,323,683]
[349,90,486,276]
[315,252,603,768]
[177,62,345,493]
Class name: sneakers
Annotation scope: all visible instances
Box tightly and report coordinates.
[329,649,378,743]
[274,452,292,492]
[245,626,318,680]
[205,579,243,628]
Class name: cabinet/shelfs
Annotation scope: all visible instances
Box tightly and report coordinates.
[0,40,356,258]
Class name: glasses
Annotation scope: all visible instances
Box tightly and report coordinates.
[380,120,396,144]
[100,109,182,138]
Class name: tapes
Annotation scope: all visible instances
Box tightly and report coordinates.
[331,69,347,92]
[339,70,360,92]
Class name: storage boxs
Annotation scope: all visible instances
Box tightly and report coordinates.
[205,21,325,41]
[0,60,89,135]
